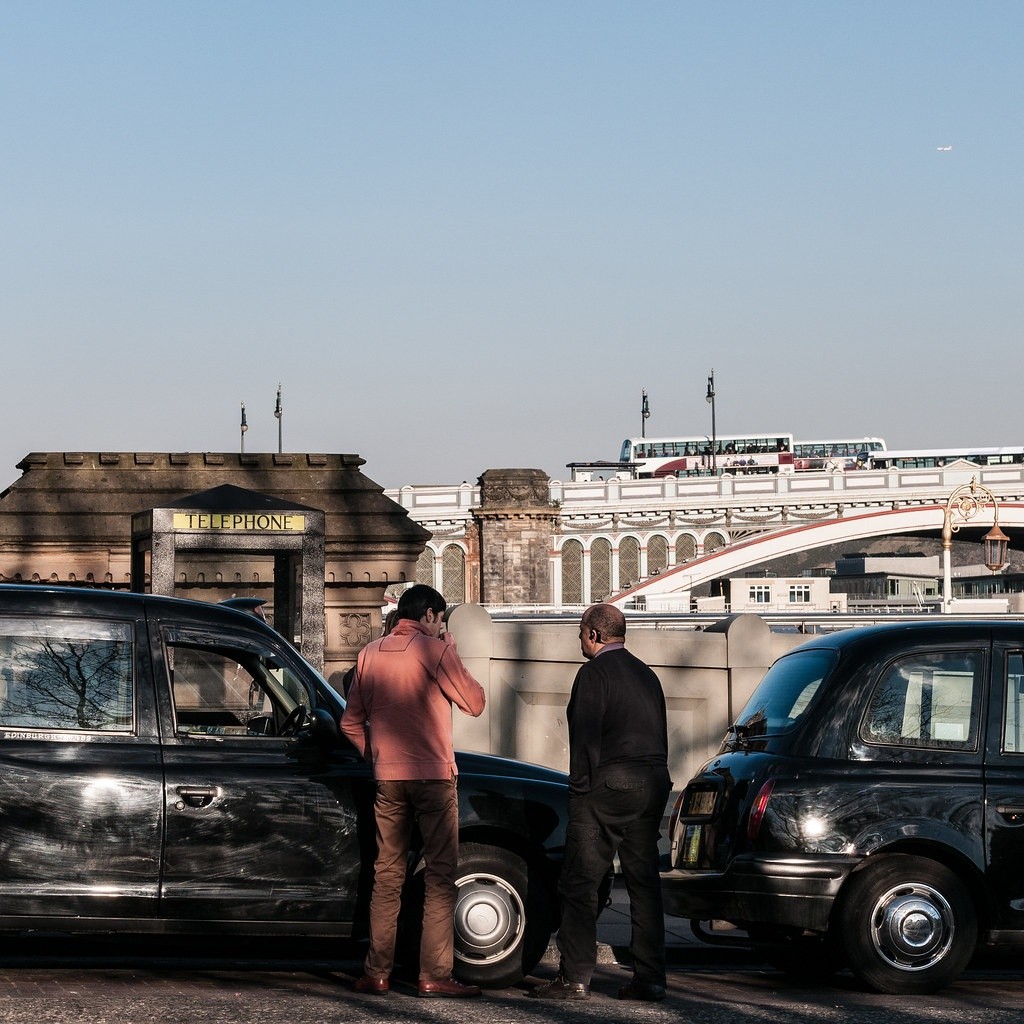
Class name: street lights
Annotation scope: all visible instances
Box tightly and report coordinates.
[706,368,718,477]
[273,382,282,453]
[940,476,1010,614]
[240,401,248,453]
[641,389,651,454]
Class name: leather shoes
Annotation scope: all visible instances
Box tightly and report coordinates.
[617,975,666,1001]
[529,974,591,1000]
[344,975,389,994]
[419,978,482,999]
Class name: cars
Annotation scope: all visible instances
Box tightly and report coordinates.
[2,583,616,994]
[654,616,1024,995]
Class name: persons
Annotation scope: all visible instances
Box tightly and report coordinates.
[794,449,859,459]
[530,602,675,1001]
[339,584,486,998]
[640,441,789,458]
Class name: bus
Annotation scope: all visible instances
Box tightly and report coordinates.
[856,447,1024,471]
[733,438,887,474]
[618,434,795,480]
